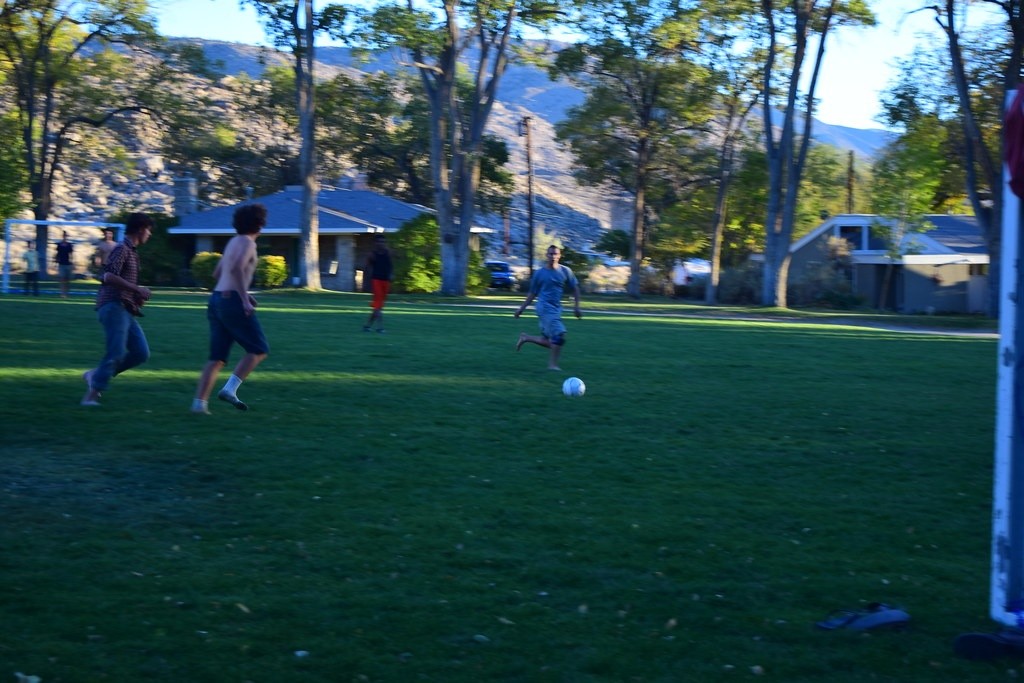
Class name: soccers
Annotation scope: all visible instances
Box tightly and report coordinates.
[562,375,587,399]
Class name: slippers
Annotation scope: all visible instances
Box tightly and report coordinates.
[815,600,912,632]
[953,630,1024,662]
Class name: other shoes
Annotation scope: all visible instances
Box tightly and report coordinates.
[362,327,387,333]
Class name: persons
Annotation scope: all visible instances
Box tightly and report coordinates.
[191,204,267,414]
[514,245,582,370]
[23,240,39,295]
[80,212,153,405]
[56,231,74,296]
[362,234,396,333]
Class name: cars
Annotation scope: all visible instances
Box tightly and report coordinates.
[485,260,518,290]
